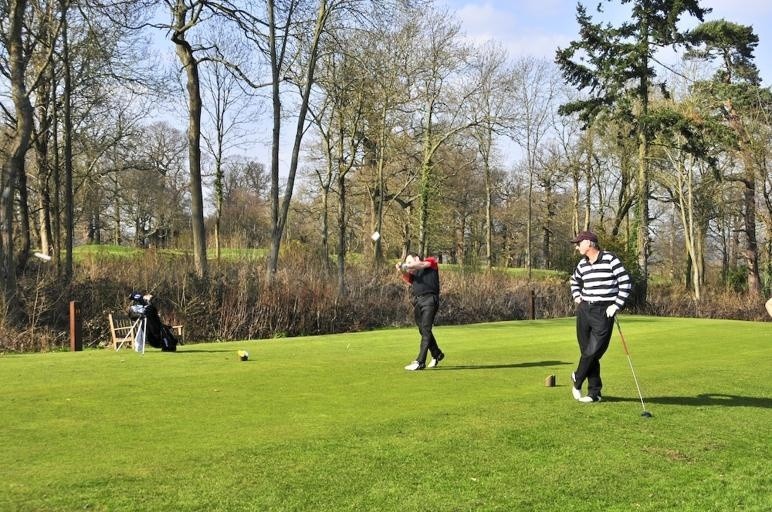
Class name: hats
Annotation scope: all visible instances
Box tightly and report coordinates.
[569,232,598,244]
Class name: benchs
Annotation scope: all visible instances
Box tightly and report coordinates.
[109,312,184,350]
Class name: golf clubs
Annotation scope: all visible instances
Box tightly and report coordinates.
[371,231,403,272]
[614,314,652,417]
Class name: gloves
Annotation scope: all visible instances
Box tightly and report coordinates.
[605,304,620,318]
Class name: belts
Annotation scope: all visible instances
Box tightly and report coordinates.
[582,300,605,305]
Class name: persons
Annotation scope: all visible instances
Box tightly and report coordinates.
[393,251,445,371]
[567,232,632,403]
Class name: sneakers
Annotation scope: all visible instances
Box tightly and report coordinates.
[403,361,427,371]
[578,395,602,403]
[571,371,583,399]
[427,352,445,369]
